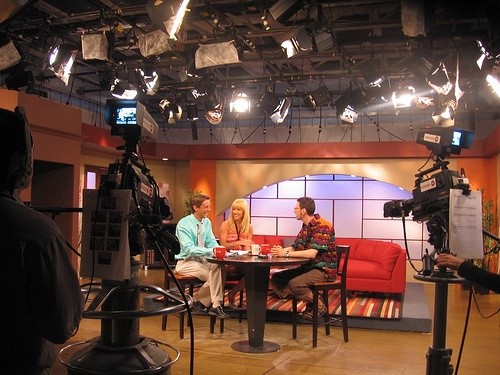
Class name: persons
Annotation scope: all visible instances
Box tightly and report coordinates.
[436,253,500,297]
[0,108,81,375]
[175,195,229,318]
[219,200,290,309]
[271,197,337,322]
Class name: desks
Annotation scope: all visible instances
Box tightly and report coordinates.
[205,255,311,353]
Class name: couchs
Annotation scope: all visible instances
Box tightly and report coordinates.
[251,234,407,294]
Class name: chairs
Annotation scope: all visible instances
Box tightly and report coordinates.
[290,244,351,348]
[162,244,244,339]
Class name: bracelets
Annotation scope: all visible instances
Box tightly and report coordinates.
[286,251,289,257]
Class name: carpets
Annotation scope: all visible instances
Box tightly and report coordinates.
[152,283,403,320]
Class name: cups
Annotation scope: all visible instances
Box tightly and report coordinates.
[250,244,262,255]
[261,243,271,255]
[213,246,226,260]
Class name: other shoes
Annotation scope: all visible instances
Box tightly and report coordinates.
[208,305,229,318]
[297,305,327,320]
[193,301,204,311]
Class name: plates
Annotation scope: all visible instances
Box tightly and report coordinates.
[230,249,249,255]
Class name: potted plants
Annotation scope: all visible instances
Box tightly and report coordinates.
[462,187,496,295]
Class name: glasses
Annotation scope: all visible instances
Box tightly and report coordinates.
[294,208,303,210]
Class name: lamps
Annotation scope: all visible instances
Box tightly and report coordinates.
[0,0,500,128]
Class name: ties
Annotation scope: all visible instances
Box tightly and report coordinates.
[197,224,205,247]
